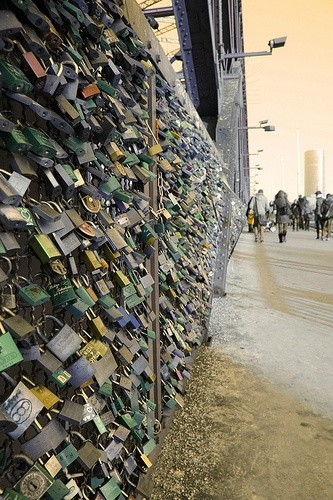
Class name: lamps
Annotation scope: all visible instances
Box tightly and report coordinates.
[248,149,263,155]
[226,36,287,59]
[249,164,263,170]
[242,120,275,132]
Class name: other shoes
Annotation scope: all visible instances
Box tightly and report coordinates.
[255,239,264,242]
[249,229,253,232]
[292,226,310,232]
[321,237,330,240]
[316,236,319,240]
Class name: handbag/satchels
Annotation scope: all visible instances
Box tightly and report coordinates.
[279,206,293,216]
[258,214,267,225]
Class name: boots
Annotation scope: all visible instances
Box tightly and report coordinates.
[279,231,286,243]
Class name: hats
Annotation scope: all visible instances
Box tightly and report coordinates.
[315,191,321,194]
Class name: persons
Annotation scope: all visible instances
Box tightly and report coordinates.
[250,188,269,242]
[312,190,325,240]
[246,194,312,232]
[322,193,333,241]
[272,190,295,242]
